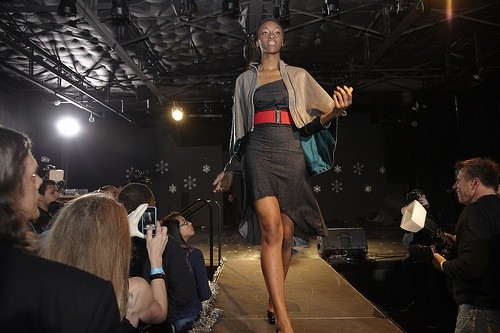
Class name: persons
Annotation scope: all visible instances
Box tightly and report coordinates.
[213,18,353,333]
[402,157,500,333]
[0,129,213,333]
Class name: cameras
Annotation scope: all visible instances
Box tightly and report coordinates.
[400,200,455,259]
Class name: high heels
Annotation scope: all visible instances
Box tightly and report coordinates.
[267,296,279,333]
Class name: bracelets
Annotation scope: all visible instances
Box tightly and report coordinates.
[150,268,164,274]
[440,259,446,272]
[150,273,165,280]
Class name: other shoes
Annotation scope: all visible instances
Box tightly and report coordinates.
[400,295,416,313]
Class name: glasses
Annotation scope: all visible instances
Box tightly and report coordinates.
[179,220,188,227]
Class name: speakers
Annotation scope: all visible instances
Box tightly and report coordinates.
[317,227,369,258]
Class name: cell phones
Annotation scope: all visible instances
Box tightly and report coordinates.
[141,206,157,236]
